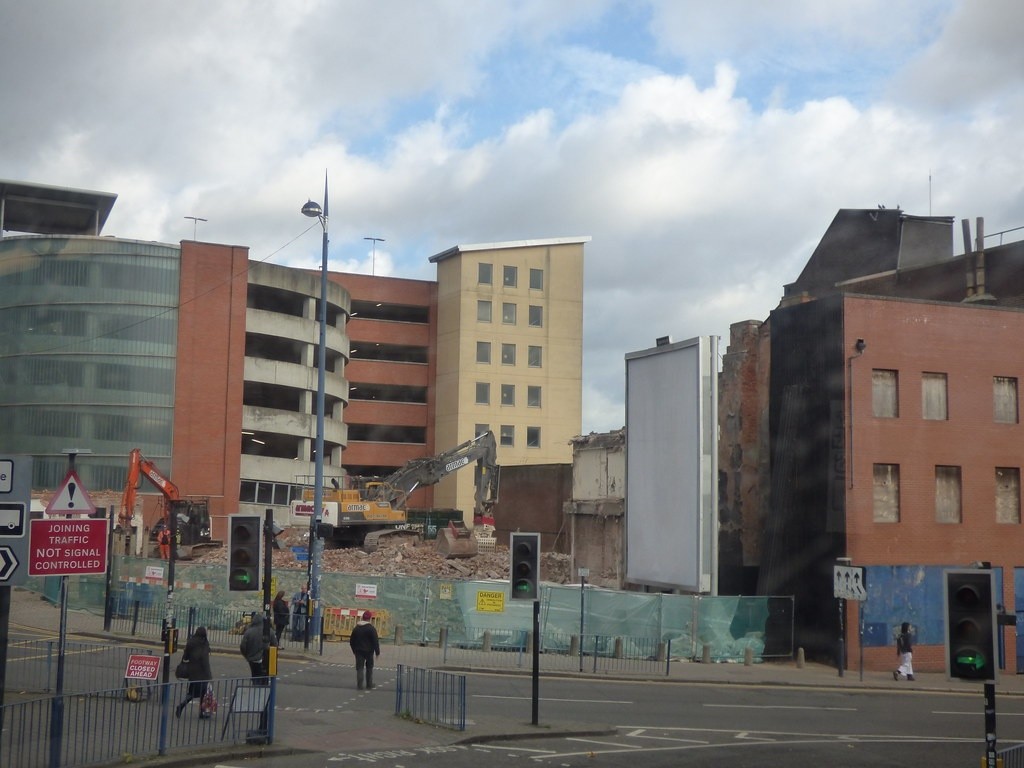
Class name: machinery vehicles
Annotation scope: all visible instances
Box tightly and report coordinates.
[290,430,501,559]
[114,448,223,560]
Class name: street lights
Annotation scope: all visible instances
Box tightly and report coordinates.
[184,217,208,242]
[301,169,329,648]
[364,237,385,276]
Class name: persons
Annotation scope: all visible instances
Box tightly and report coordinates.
[176,626,211,719]
[349,609,380,691]
[893,622,916,681]
[240,614,279,685]
[290,585,307,642]
[274,590,290,650]
[158,525,170,559]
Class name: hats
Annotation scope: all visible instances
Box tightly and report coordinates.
[362,610,372,621]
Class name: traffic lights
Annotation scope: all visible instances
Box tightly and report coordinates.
[943,569,999,684]
[88,507,106,518]
[228,514,262,593]
[510,532,540,602]
[318,523,333,537]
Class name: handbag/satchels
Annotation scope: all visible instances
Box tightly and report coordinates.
[201,682,217,716]
[175,655,190,679]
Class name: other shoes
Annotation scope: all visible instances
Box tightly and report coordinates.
[357,686,364,690]
[175,704,181,718]
[199,714,210,719]
[277,646,284,650]
[366,683,375,689]
